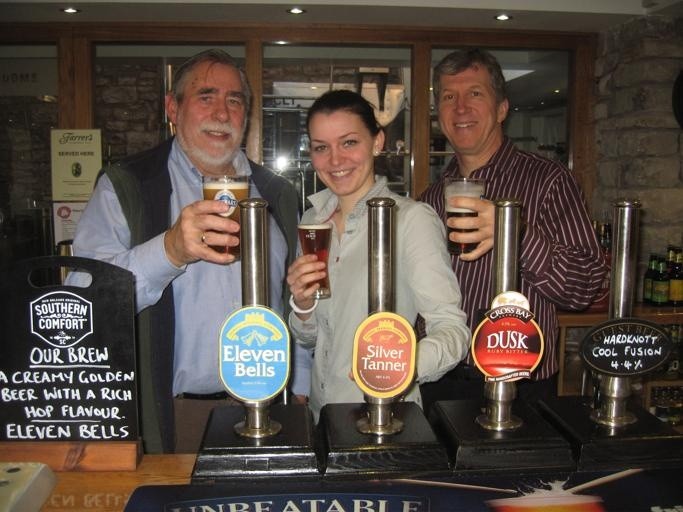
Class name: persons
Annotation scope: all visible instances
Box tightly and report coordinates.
[284,87,472,429]
[56,43,311,453]
[414,48,607,403]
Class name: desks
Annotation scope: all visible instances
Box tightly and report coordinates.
[1,452,681,512]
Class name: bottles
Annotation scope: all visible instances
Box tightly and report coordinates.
[641,244,683,307]
[649,323,683,427]
[590,218,611,311]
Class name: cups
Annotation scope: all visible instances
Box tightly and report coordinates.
[441,176,488,254]
[199,176,251,259]
[294,224,332,299]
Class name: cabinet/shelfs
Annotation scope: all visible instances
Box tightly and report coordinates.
[557,302,683,427]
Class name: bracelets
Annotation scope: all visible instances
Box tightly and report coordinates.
[288,291,325,316]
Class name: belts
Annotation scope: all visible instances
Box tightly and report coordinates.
[176,389,230,403]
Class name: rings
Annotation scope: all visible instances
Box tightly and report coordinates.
[200,234,206,244]
